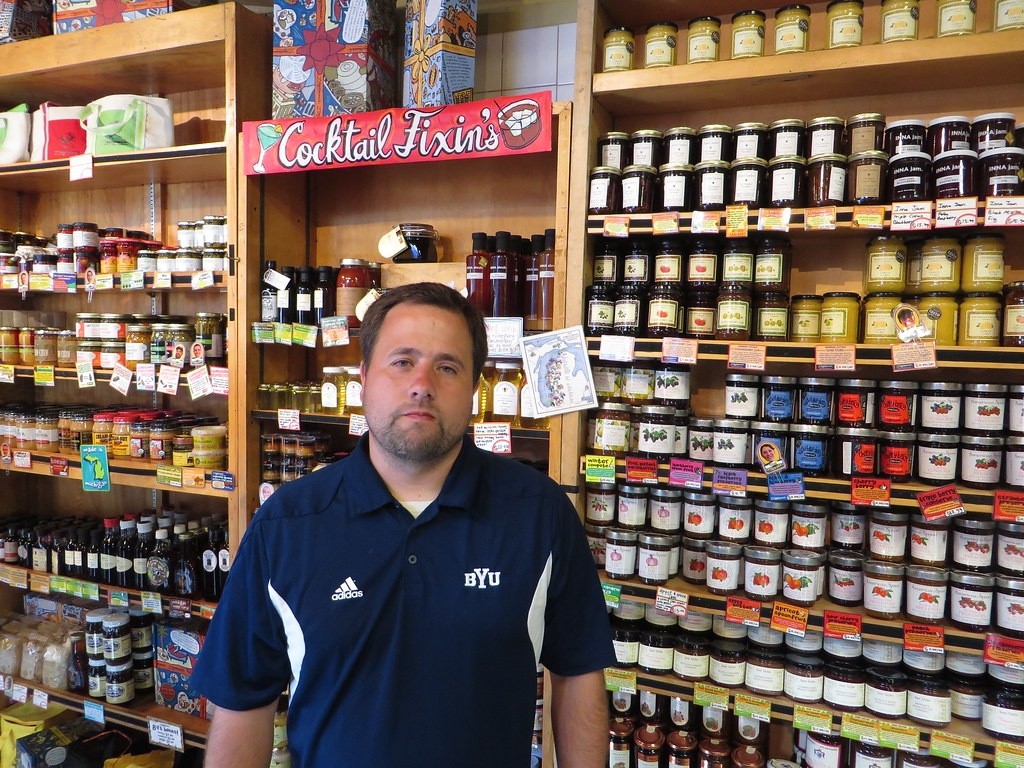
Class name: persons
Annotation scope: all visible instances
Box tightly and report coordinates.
[898,310,915,327]
[186,281,619,768]
[176,347,183,359]
[2,444,9,456]
[21,274,27,286]
[192,344,203,361]
[87,270,93,283]
[761,444,775,462]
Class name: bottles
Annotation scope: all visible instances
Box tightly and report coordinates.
[68,628,88,693]
[0,503,229,601]
[260,260,341,327]
[466,229,555,335]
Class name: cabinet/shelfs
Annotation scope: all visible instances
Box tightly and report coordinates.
[0,0,1023,768]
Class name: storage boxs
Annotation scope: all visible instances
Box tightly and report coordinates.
[0,702,180,768]
[273,0,394,121]
[0,0,49,45]
[52,0,170,36]
[402,0,477,109]
